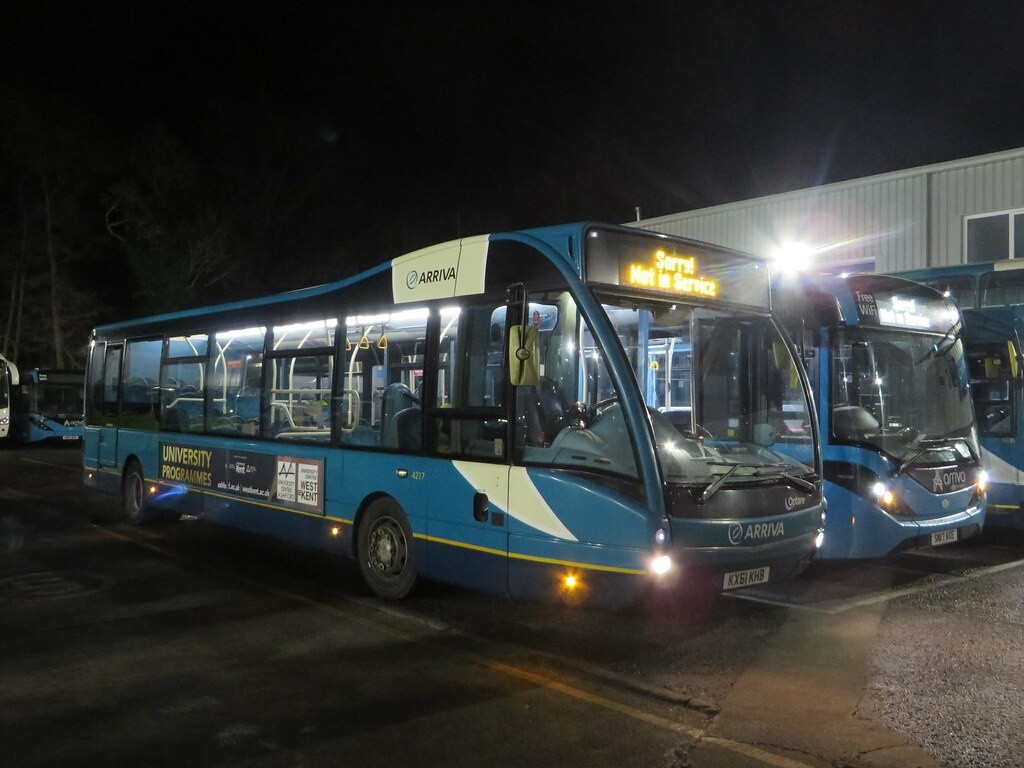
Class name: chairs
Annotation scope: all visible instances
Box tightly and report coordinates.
[377,383,411,448]
[529,375,568,445]
[97,374,378,450]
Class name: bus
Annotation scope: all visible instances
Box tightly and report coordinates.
[869,254,1024,531]
[580,268,1024,576]
[82,218,830,613]
[0,352,20,440]
[15,367,87,446]
